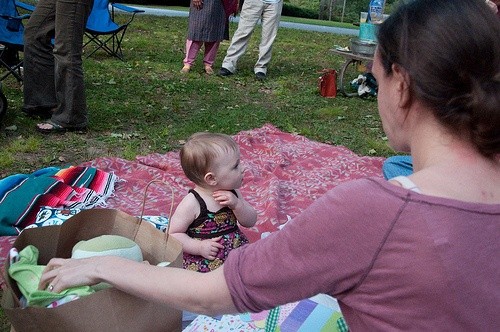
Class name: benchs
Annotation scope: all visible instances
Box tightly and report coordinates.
[328,46,374,97]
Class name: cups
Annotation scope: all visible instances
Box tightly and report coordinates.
[360,12,368,23]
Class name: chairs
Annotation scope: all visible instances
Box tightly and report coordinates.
[0,0,57,92]
[81,0,146,62]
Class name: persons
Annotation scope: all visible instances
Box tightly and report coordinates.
[38,0,500,332]
[219,0,283,80]
[165,132,257,272]
[179,0,229,75]
[21,0,94,134]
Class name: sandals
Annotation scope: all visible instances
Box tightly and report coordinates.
[21,105,53,119]
[35,119,87,135]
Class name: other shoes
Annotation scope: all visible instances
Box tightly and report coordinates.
[203,65,214,75]
[219,68,234,76]
[255,72,267,81]
[180,65,190,73]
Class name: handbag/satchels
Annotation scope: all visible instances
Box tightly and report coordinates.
[1,179,184,332]
[317,69,338,99]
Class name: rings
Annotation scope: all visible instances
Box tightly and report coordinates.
[47,284,54,292]
